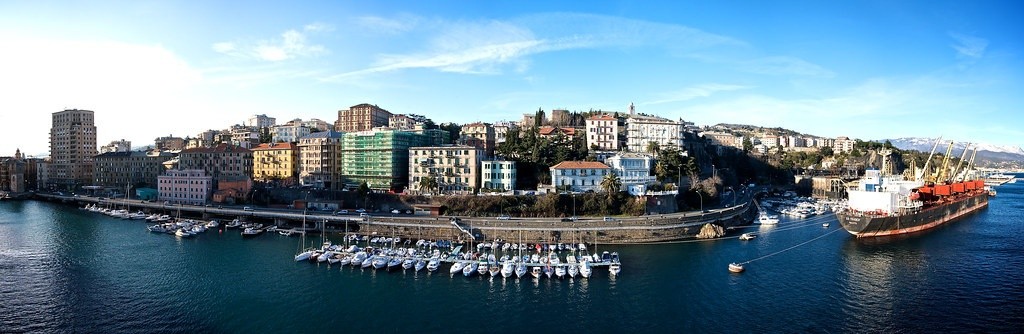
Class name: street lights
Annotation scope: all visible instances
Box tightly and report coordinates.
[712,165,714,180]
[728,187,735,206]
[696,191,702,211]
[573,195,575,218]
[678,167,680,187]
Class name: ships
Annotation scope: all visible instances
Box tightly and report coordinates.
[835,136,988,246]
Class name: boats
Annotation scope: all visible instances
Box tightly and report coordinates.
[84,180,219,238]
[982,168,1016,197]
[295,210,622,278]
[753,197,850,225]
[226,219,297,236]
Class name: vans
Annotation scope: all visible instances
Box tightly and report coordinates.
[604,217,615,221]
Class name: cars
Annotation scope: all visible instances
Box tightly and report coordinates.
[338,210,348,214]
[244,207,254,211]
[497,215,511,220]
[391,210,401,214]
[356,208,370,217]
